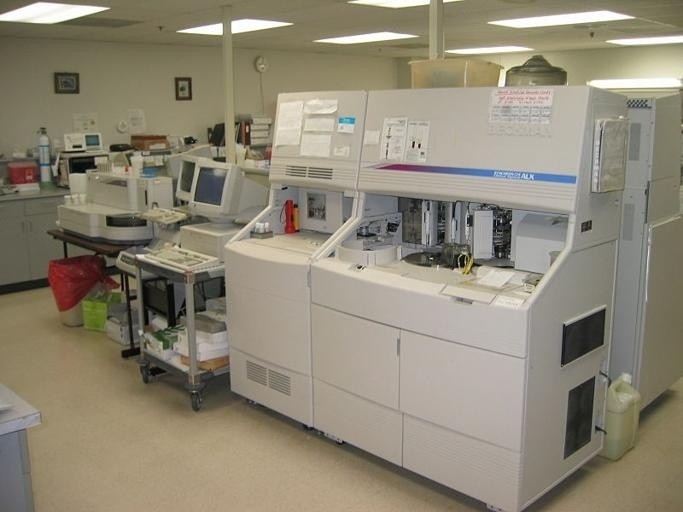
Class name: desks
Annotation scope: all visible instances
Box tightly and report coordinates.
[46,228,139,358]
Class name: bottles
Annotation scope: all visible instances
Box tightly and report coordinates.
[292,204,300,231]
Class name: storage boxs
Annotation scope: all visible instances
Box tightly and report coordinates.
[105,317,139,346]
[407,56,504,89]
[81,292,121,332]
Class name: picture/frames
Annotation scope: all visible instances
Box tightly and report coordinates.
[174,76,192,101]
[53,71,80,94]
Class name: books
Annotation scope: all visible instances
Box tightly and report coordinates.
[209,121,239,146]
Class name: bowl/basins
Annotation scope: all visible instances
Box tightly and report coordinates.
[141,168,159,177]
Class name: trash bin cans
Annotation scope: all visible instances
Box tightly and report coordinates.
[49,255,107,326]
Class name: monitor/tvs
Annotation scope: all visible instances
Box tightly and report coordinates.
[176,155,213,202]
[189,161,268,225]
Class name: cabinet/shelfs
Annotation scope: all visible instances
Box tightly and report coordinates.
[0,194,66,293]
[130,252,229,412]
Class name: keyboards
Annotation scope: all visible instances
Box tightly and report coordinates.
[136,246,219,272]
[136,207,187,224]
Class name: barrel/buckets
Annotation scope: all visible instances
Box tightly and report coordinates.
[601,373,638,461]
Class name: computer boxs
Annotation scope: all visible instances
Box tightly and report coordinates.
[180,223,243,259]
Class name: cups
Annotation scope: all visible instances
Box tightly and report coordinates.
[130,155,143,178]
